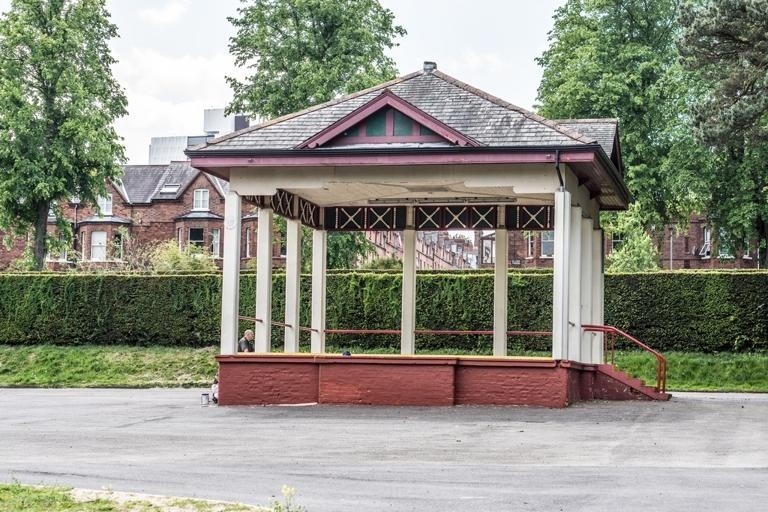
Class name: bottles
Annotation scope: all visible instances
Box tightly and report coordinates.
[201,392,209,408]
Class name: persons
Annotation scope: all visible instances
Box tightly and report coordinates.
[211,366,218,404]
[238,329,255,352]
